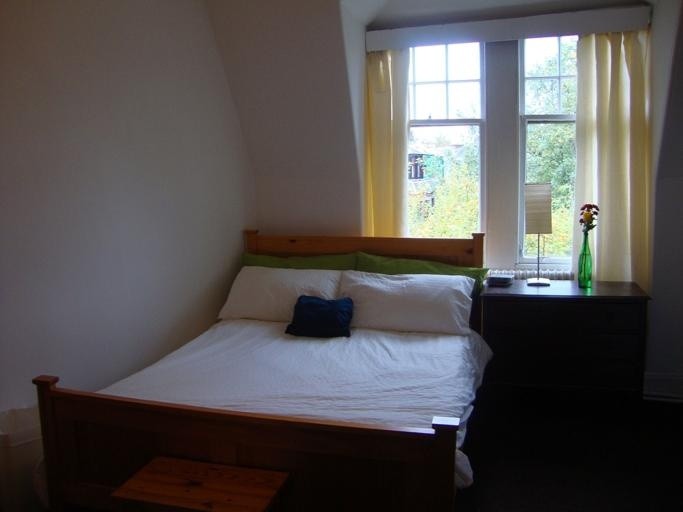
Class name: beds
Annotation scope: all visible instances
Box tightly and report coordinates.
[29,226,485,512]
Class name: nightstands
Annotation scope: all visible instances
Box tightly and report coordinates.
[477,277,652,428]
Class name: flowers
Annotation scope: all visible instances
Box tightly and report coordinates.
[578,203,600,280]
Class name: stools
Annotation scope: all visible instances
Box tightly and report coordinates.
[108,456,294,511]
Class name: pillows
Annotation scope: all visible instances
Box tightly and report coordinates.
[214,252,489,339]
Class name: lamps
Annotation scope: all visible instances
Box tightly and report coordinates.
[524,183,553,286]
